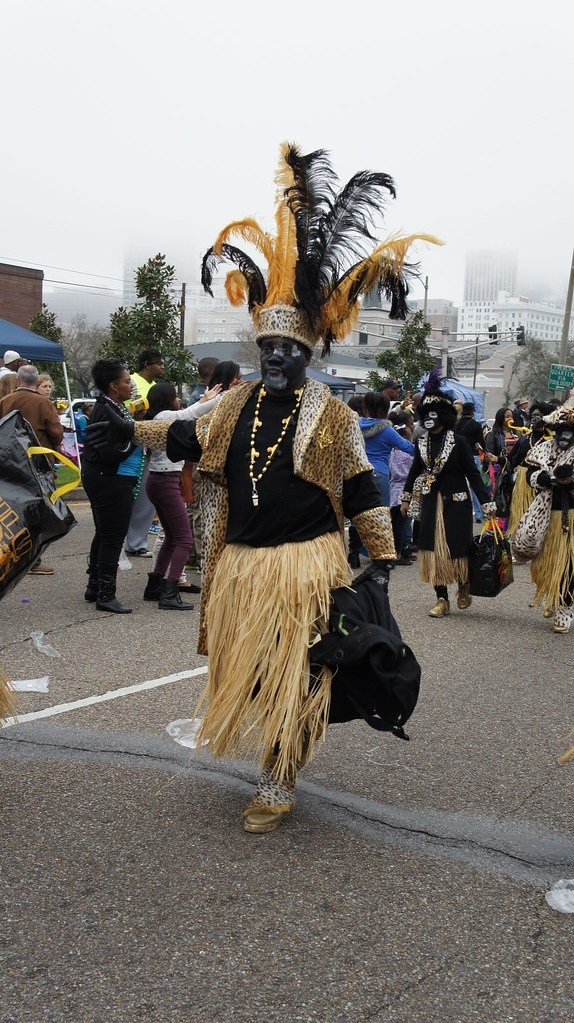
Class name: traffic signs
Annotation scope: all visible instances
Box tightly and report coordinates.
[548,363,574,391]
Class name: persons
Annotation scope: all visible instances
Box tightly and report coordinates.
[83,306,398,831]
[0,349,574,632]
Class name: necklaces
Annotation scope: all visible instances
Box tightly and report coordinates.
[102,394,149,500]
[249,382,306,508]
[427,431,446,471]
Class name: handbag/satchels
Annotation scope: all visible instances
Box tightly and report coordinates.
[467,515,514,597]
[0,410,84,601]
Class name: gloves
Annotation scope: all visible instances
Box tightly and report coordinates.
[537,471,552,487]
[350,560,395,596]
[85,402,135,449]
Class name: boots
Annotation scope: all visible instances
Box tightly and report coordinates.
[96,564,133,615]
[158,578,194,609]
[84,555,98,601]
[143,572,166,601]
[347,526,363,570]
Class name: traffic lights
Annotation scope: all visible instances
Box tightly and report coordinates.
[488,324,498,345]
[516,325,526,345]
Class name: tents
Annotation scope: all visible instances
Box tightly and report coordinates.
[0,318,81,471]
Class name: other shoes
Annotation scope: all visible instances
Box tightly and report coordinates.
[454,582,472,608]
[175,583,202,595]
[125,547,153,558]
[543,610,555,618]
[428,597,451,619]
[244,806,284,834]
[27,564,54,574]
[391,542,419,566]
[148,525,162,534]
[553,624,569,633]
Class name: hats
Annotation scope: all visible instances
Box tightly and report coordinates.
[139,348,164,370]
[4,350,20,365]
[460,402,476,412]
[384,378,403,390]
[519,399,529,405]
[201,143,440,352]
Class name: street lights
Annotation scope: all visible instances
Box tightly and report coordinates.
[403,266,428,330]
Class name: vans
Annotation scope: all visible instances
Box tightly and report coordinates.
[57,398,97,430]
